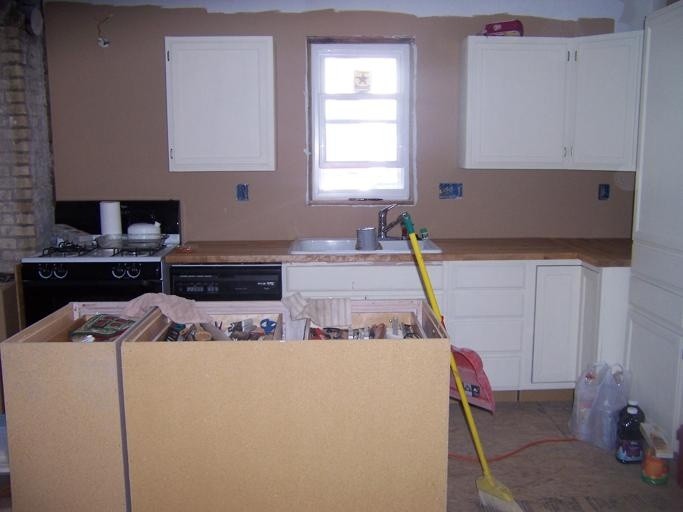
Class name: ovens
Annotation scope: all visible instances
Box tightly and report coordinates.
[169,262,282,301]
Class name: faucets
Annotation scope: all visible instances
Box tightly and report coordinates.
[378,203,401,239]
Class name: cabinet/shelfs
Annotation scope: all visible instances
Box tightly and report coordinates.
[625,2,683,452]
[455,29,645,171]
[164,35,278,172]
[281,255,631,403]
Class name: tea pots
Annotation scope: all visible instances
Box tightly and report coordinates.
[126,221,162,250]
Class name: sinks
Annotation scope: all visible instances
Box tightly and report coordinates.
[288,238,442,256]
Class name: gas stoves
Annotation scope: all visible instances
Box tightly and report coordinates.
[20,242,178,282]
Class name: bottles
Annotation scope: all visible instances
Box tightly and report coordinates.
[616,401,644,464]
[641,445,670,486]
[420,227,430,241]
[572,373,626,450]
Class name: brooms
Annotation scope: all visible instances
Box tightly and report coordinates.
[402,214,522,512]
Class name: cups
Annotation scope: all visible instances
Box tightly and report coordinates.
[355,227,380,250]
